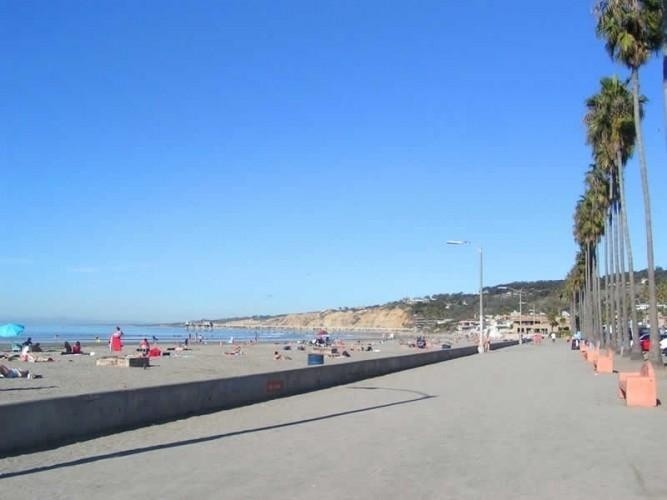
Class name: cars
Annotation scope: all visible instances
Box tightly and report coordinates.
[601,325,666,356]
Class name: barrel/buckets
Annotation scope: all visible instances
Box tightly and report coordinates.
[442,344,451,348]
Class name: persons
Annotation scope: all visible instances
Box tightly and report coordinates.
[72,340,90,355]
[20,342,52,362]
[198,334,202,343]
[0,363,42,380]
[135,349,151,358]
[32,343,41,351]
[95,335,101,343]
[26,337,33,345]
[188,333,192,345]
[270,333,493,361]
[139,338,150,349]
[550,332,557,344]
[110,326,122,352]
[184,338,191,349]
[60,340,72,355]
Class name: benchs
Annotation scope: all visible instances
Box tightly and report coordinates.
[593,348,613,372]
[617,360,657,407]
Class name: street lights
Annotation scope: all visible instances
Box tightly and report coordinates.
[527,308,542,334]
[517,302,535,337]
[497,287,523,344]
[446,240,484,355]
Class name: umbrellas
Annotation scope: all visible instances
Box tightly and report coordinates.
[313,328,330,340]
[0,322,24,348]
